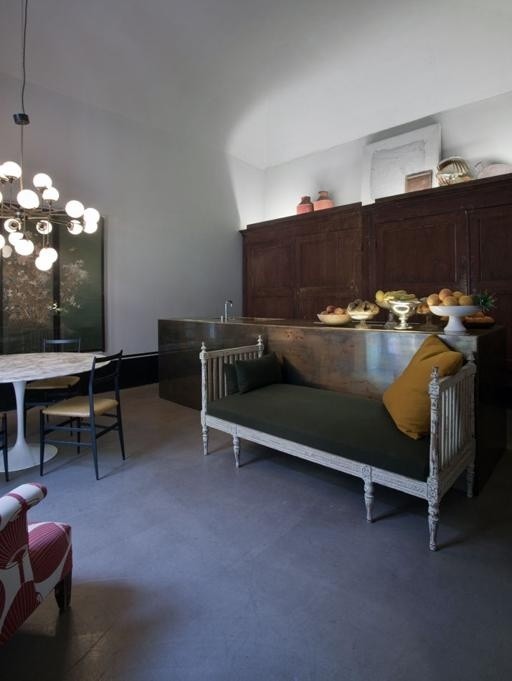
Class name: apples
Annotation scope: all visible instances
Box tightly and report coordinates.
[427,289,474,306]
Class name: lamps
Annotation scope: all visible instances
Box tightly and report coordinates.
[0,0,100,272]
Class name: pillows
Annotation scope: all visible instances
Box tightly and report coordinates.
[224,363,238,394]
[236,352,281,395]
[383,334,464,440]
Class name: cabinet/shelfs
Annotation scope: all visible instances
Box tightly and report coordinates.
[240,172,512,409]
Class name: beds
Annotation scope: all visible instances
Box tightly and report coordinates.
[199,336,477,551]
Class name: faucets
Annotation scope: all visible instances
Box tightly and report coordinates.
[219,299,233,321]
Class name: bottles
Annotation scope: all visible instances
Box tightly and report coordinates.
[224,300,233,321]
[313,191,333,211]
[297,196,313,215]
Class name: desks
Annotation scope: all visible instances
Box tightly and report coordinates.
[0,352,111,474]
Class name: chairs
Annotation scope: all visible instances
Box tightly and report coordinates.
[0,482,72,649]
[23,336,81,438]
[0,410,10,481]
[39,350,126,480]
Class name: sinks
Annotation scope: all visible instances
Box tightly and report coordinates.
[212,315,285,324]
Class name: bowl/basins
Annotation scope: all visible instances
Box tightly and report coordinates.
[316,313,350,325]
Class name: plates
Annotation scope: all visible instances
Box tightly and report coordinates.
[431,316,497,330]
